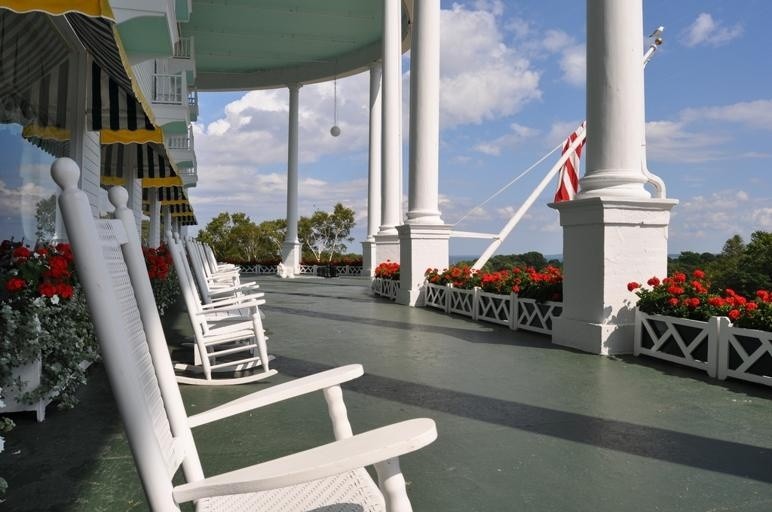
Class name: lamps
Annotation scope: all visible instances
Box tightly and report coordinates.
[329,51,343,137]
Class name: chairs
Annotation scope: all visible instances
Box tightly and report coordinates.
[51,156,441,510]
[153,224,284,390]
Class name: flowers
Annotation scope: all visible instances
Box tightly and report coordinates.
[1,228,179,418]
[441,253,771,358]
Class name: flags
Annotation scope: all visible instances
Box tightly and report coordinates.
[553,131,586,203]
